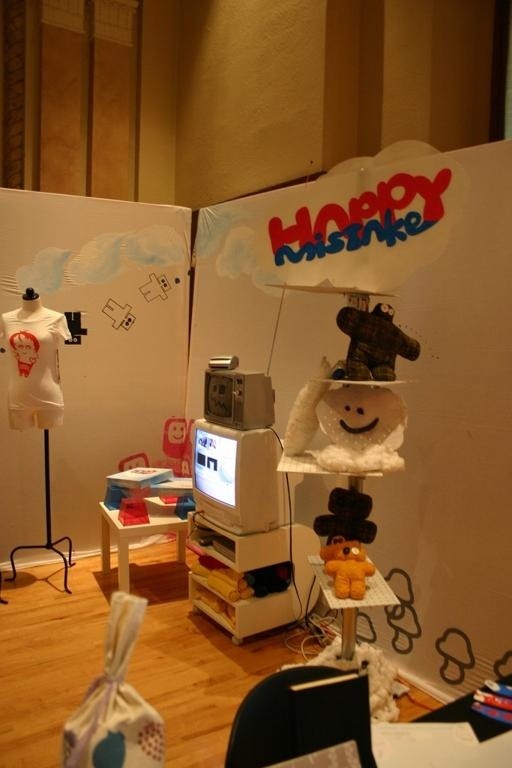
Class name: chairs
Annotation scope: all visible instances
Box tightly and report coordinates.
[223,664,353,768]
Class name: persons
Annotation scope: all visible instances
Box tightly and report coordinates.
[0,287,72,430]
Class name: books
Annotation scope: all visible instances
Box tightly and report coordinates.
[105,465,193,517]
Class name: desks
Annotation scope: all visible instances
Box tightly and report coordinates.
[98,498,189,596]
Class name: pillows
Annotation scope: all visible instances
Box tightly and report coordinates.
[282,354,334,458]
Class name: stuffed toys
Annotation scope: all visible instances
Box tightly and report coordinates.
[336,303,421,382]
[319,540,375,600]
[313,487,377,545]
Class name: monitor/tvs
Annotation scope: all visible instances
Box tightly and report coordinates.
[192,418,304,536]
[203,368,275,431]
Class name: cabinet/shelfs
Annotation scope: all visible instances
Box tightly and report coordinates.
[184,510,321,646]
